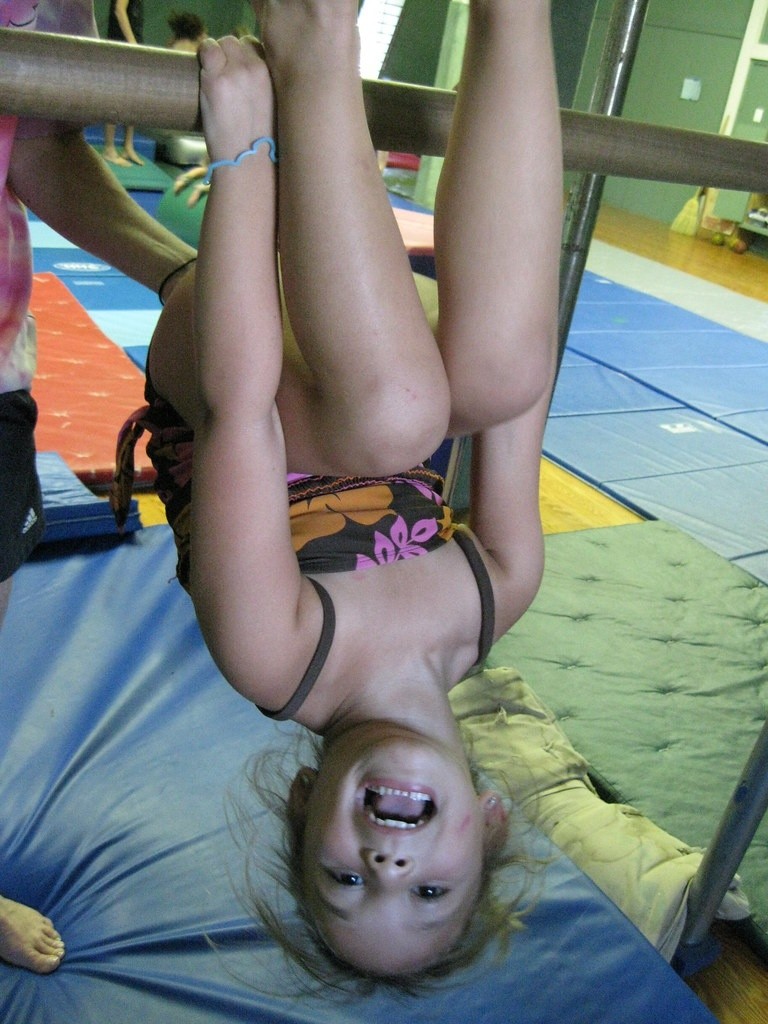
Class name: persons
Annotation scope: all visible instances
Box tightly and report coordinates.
[146,0,567,1005]
[0,0,201,975]
[164,12,210,54]
[102,0,143,166]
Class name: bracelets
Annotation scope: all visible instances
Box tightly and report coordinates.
[158,258,198,308]
[202,137,280,187]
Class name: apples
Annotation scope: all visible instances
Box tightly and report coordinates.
[711,233,747,253]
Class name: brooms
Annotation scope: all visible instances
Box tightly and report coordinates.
[669,114,731,235]
